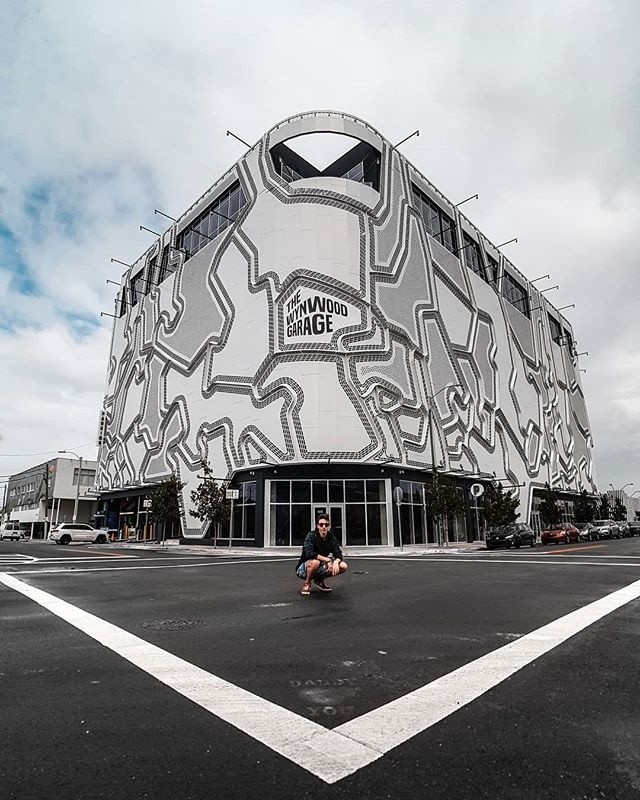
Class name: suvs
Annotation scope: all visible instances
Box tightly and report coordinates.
[0,522,21,541]
[49,522,109,545]
[590,519,640,539]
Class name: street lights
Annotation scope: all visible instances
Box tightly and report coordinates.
[58,450,83,524]
[630,491,640,506]
[620,483,633,503]
[610,484,615,510]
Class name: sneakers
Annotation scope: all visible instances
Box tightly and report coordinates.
[314,578,336,591]
[301,581,311,594]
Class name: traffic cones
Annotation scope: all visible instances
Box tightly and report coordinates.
[110,533,115,541]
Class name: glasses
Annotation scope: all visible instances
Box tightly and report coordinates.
[318,522,331,528]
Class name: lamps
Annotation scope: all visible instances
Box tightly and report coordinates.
[558,304,575,311]
[76,477,162,497]
[496,238,518,248]
[197,474,229,481]
[100,208,235,319]
[553,335,588,357]
[511,296,543,313]
[260,460,275,465]
[432,224,475,253]
[541,285,558,292]
[530,274,550,282]
[376,459,395,466]
[226,130,252,149]
[394,129,419,148]
[474,261,509,283]
[428,460,525,489]
[456,194,479,206]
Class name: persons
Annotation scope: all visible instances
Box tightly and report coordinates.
[296,514,347,594]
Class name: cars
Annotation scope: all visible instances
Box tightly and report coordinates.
[486,523,536,550]
[541,523,580,546]
[573,522,600,542]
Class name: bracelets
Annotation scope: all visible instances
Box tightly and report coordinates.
[326,561,330,563]
